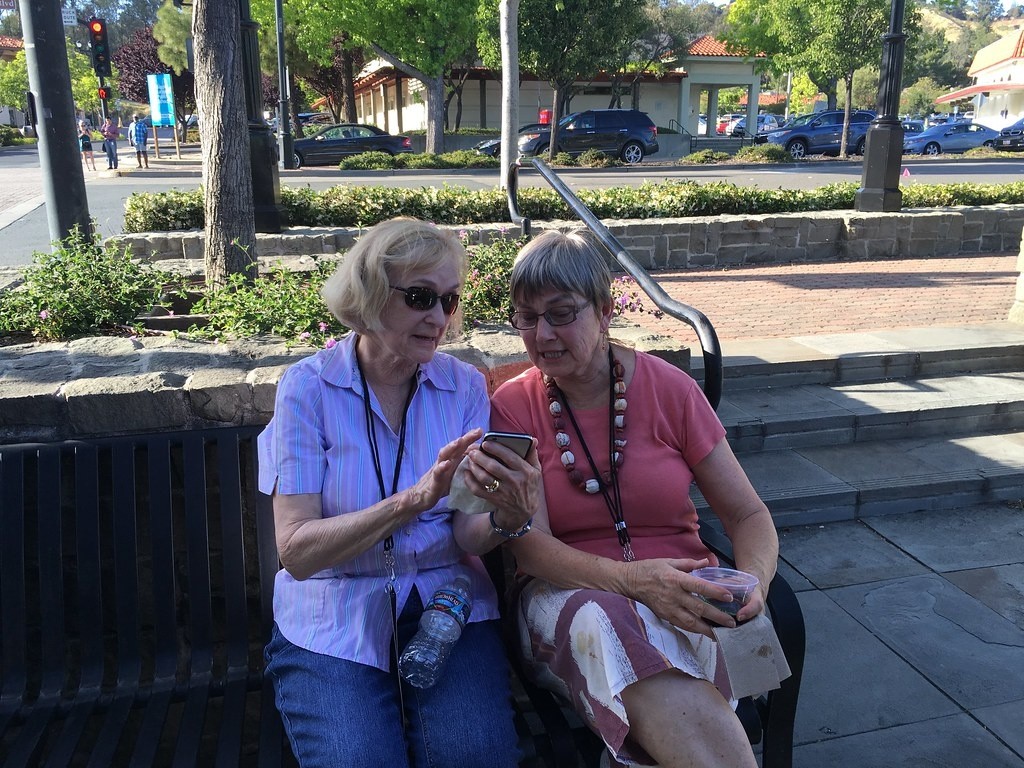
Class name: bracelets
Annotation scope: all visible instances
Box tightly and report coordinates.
[489,509,534,538]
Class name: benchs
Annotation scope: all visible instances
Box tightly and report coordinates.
[0,425,805,767]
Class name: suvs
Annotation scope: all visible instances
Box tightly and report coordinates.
[518,108,659,164]
[732,115,778,138]
[288,113,344,131]
[754,110,875,159]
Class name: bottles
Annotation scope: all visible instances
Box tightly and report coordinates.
[398,574,473,690]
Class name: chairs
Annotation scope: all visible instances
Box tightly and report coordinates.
[342,130,351,138]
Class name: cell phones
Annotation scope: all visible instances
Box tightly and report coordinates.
[479,433,533,470]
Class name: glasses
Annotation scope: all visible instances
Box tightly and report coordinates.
[507,299,592,330]
[386,284,461,316]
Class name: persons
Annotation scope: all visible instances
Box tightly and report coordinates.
[490,228,779,768]
[76,120,97,171]
[127,114,149,168]
[101,116,120,169]
[258,215,542,768]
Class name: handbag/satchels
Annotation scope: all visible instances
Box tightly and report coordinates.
[82,141,91,149]
[102,144,106,152]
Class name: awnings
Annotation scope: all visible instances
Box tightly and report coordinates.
[935,84,1024,105]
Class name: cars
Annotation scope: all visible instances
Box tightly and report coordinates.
[178,115,196,126]
[293,123,414,169]
[699,114,740,133]
[773,116,785,127]
[140,114,152,127]
[902,123,1000,156]
[901,121,923,137]
[926,112,973,128]
[471,123,550,160]
[992,118,1024,152]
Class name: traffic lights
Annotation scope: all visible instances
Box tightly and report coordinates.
[99,87,111,99]
[88,19,111,77]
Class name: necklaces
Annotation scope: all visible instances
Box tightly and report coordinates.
[542,347,635,563]
[355,348,419,595]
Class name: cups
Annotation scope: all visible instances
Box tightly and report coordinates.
[692,567,759,627]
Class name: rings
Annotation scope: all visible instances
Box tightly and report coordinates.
[484,479,500,494]
